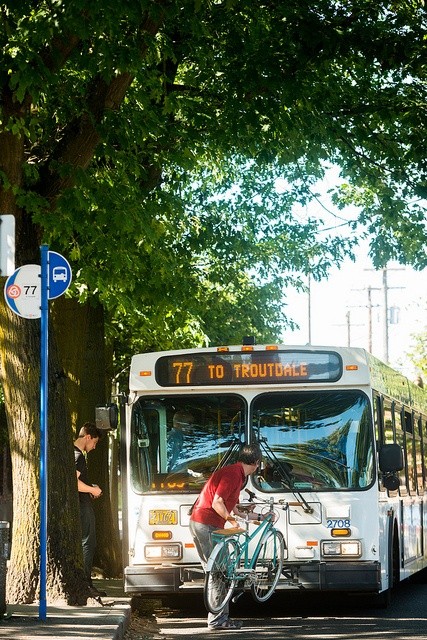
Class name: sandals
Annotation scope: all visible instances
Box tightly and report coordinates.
[208,620,240,629]
[228,618,243,626]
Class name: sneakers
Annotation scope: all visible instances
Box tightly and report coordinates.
[88,585,107,597]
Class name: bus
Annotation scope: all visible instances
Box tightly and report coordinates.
[94,343,427,617]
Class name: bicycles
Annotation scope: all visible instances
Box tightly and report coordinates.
[202,502,314,617]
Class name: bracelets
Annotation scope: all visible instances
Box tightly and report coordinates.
[258,514,260,521]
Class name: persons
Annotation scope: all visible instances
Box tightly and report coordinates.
[189,444,276,629]
[157,411,194,474]
[309,419,359,487]
[73,422,108,597]
